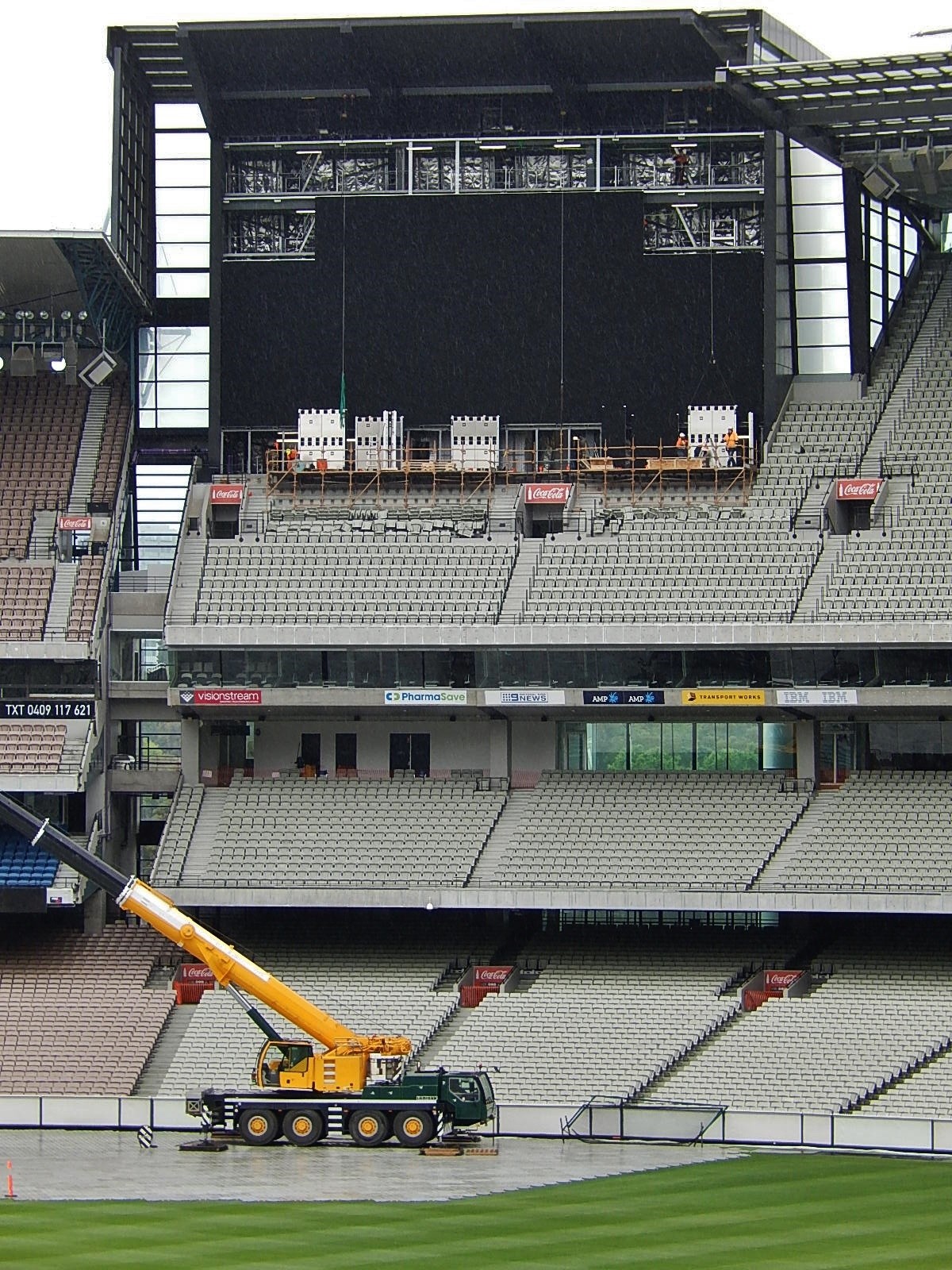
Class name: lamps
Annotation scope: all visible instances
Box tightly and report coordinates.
[0,309,87,322]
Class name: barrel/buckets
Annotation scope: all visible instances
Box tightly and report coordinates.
[317,459,327,470]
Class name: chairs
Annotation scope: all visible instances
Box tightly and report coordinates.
[192,248,952,623]
[0,371,134,641]
[150,769,952,893]
[0,723,67,887]
[0,919,952,1119]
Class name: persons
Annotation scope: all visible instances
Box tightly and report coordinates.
[286,448,299,471]
[675,432,688,459]
[720,426,739,467]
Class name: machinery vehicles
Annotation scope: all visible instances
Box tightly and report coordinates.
[0,795,500,1152]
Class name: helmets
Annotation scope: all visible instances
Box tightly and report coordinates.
[728,426,733,431]
[680,432,686,437]
